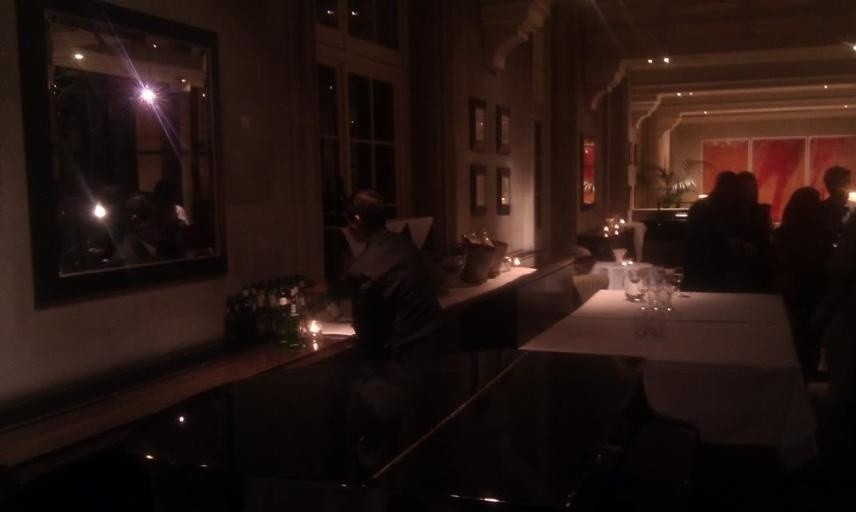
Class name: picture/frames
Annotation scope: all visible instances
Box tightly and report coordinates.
[468,95,513,217]
[14,2,232,313]
[579,133,599,210]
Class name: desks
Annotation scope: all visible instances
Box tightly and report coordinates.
[515,288,807,511]
[593,261,655,284]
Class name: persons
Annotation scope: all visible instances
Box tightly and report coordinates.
[806,215,856,411]
[820,165,856,249]
[57,176,191,265]
[686,168,737,247]
[751,186,829,390]
[341,188,460,483]
[710,168,774,292]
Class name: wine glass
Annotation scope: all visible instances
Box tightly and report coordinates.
[624,262,689,340]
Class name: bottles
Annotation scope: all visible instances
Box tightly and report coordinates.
[223,272,310,349]
